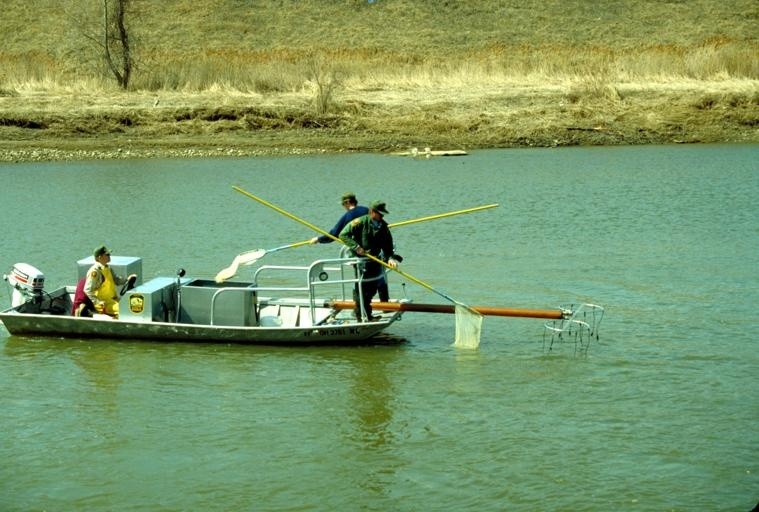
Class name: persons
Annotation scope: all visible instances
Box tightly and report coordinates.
[84,246,129,318]
[311,192,393,313]
[341,201,398,322]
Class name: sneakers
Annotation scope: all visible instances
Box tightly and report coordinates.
[356,312,382,323]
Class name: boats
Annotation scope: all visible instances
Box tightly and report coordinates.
[0,244,412,341]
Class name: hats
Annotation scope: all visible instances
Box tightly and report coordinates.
[370,200,390,216]
[337,192,358,204]
[94,245,113,258]
